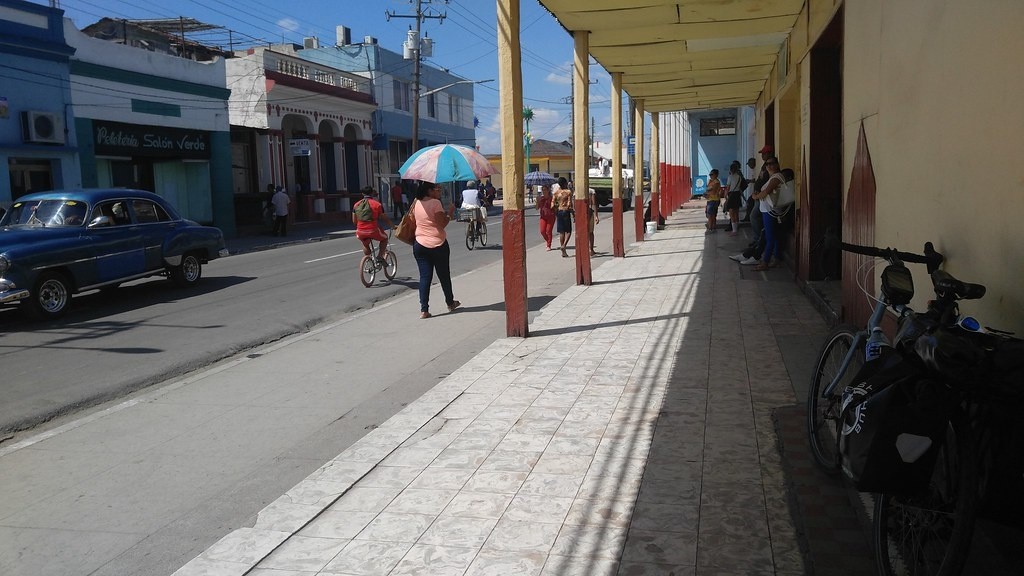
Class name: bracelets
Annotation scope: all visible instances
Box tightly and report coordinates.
[446,214,451,221]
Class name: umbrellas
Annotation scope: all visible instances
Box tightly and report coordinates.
[524,171,558,197]
[398,143,495,204]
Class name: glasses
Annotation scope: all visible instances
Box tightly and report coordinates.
[709,174,713,176]
[765,162,775,166]
[431,188,442,192]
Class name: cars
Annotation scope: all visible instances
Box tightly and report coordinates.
[0,188,229,322]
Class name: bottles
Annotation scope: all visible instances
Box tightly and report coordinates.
[866,327,891,362]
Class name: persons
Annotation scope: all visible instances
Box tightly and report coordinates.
[413,182,461,318]
[703,145,794,270]
[353,186,398,266]
[644,200,664,232]
[262,184,291,237]
[390,179,418,217]
[536,177,599,256]
[65,207,115,225]
[459,181,496,237]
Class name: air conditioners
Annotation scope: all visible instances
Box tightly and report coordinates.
[19,110,65,145]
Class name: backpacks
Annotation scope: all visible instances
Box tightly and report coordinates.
[355,199,374,222]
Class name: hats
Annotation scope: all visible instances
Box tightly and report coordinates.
[759,145,774,153]
[467,181,475,188]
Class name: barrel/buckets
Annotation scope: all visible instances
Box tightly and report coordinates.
[646,222,657,233]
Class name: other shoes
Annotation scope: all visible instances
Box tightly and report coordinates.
[448,301,461,312]
[729,252,749,261]
[705,218,748,231]
[751,264,769,271]
[740,256,760,265]
[420,312,431,319]
[590,249,596,256]
[561,246,568,257]
[377,256,388,267]
[546,247,551,251]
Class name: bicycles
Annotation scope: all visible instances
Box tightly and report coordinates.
[807,240,1024,576]
[465,204,487,250]
[359,228,397,287]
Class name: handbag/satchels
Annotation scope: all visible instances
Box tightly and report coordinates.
[740,179,748,191]
[838,349,944,497]
[395,215,415,245]
[767,173,794,208]
[723,200,728,213]
